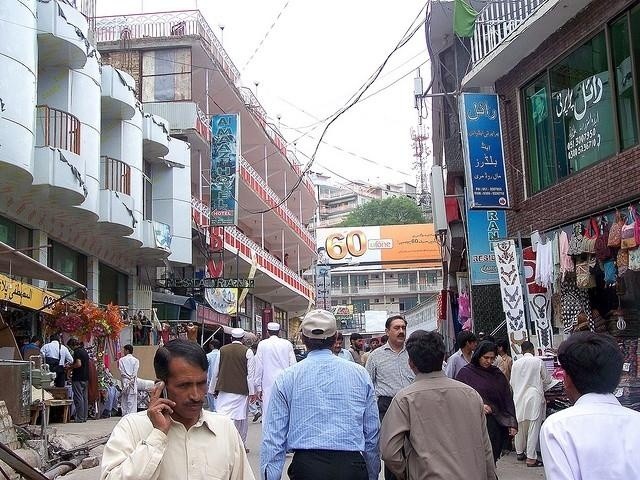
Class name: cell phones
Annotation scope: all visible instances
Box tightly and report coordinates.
[154,379,169,415]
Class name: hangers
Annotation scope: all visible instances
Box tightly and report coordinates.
[534,195,639,246]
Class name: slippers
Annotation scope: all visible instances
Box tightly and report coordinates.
[517,454,526,460]
[527,460,543,466]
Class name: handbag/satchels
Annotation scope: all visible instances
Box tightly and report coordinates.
[43,357,59,372]
[566,207,640,288]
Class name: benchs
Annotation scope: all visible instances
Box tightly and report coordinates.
[43,396,74,425]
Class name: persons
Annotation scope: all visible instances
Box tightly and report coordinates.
[437,332,551,467]
[367,316,417,478]
[259,307,382,479]
[283,253,288,266]
[101,337,255,480]
[538,331,640,480]
[23,334,90,423]
[379,330,497,479]
[118,344,140,417]
[206,322,297,453]
[336,333,389,369]
[317,247,329,264]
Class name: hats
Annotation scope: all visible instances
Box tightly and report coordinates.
[232,328,245,338]
[267,322,280,330]
[303,309,336,339]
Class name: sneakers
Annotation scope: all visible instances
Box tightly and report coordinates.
[253,412,262,421]
